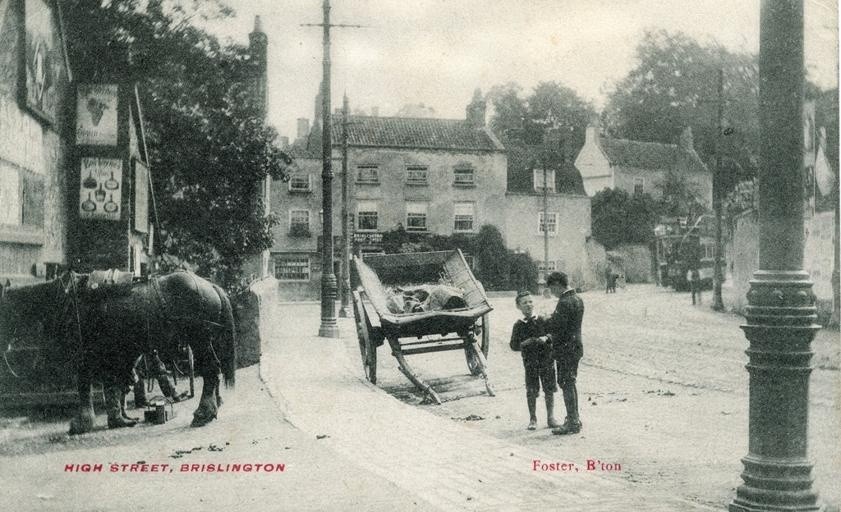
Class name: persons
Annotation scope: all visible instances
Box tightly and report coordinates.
[605,265,619,293]
[510,292,559,430]
[543,271,583,434]
[687,261,703,305]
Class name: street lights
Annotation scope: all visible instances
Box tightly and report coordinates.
[712,44,731,310]
[541,157,548,295]
[300,0,366,338]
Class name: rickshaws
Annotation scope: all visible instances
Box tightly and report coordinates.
[351,249,495,404]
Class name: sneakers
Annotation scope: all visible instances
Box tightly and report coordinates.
[528,418,535,429]
[548,416,582,434]
[136,401,150,406]
[174,391,188,401]
[108,413,139,428]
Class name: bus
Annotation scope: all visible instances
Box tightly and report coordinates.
[668,240,725,291]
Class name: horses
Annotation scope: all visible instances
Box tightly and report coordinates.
[0,268,235,436]
